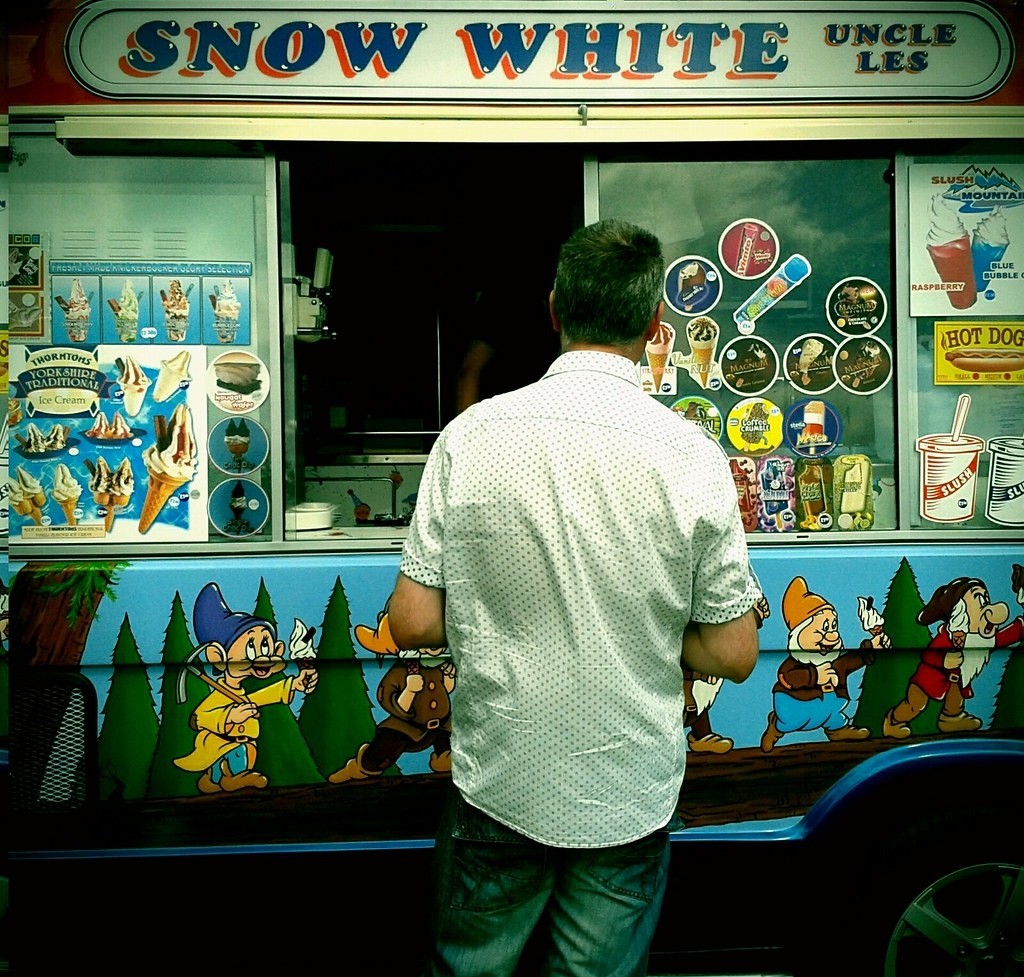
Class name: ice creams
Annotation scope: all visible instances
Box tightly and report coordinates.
[6,279,250,534]
[646,323,674,393]
[687,317,718,389]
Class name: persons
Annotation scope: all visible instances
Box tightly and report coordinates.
[388,219,762,977]
[455,260,562,418]
